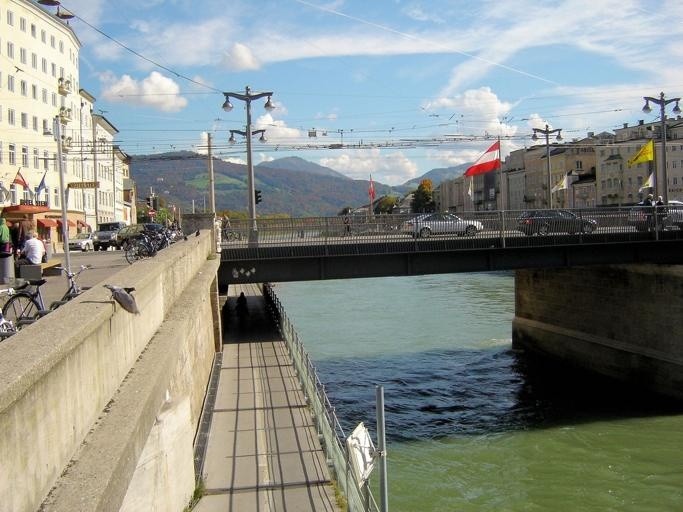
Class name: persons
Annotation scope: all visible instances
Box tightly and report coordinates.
[342,215,352,237]
[642,194,654,231]
[12,228,48,275]
[654,196,667,231]
[220,300,233,333]
[222,214,230,240]
[235,291,250,320]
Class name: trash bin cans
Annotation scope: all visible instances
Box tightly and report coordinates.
[0,252,15,283]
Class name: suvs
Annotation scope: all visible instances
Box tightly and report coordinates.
[92,221,125,251]
[628,201,682,230]
[515,209,598,235]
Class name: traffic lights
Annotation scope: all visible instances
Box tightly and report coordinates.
[255,188,264,205]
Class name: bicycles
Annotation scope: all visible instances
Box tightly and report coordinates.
[124,225,184,267]
[0,262,94,345]
[221,223,247,241]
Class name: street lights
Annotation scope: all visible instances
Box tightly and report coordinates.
[222,85,279,248]
[227,124,269,147]
[532,124,564,210]
[643,91,683,229]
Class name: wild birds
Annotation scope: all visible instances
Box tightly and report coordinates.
[184,236,187,241]
[148,247,157,257]
[103,284,140,316]
[196,228,200,236]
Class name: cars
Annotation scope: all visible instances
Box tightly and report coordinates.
[63,232,94,252]
[402,210,483,239]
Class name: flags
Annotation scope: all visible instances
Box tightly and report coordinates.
[638,172,655,193]
[463,141,500,178]
[551,175,567,195]
[625,137,653,169]
[13,171,28,192]
[468,176,475,201]
[368,177,375,200]
[36,174,47,195]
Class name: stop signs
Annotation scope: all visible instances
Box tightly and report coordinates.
[148,209,156,217]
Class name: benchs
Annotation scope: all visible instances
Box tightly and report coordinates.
[19,259,63,282]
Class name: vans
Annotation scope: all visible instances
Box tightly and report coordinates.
[111,222,163,251]
